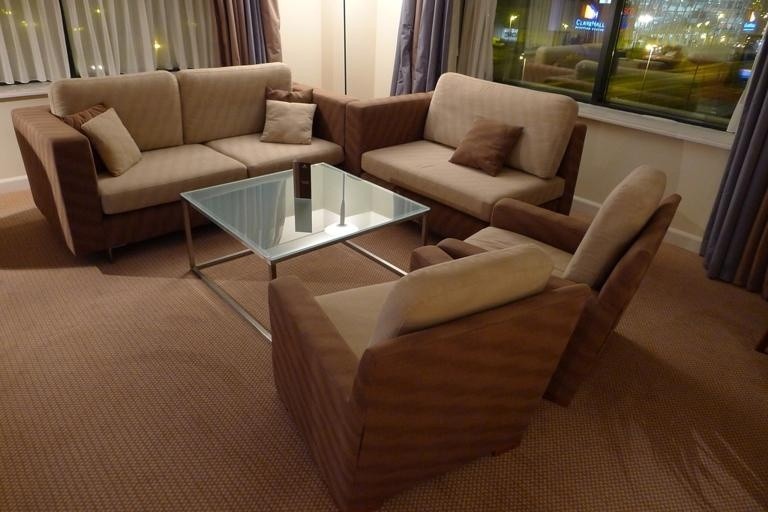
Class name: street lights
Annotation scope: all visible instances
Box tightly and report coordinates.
[686,21,710,106]
[520,53,526,81]
[639,44,658,101]
[509,16,517,36]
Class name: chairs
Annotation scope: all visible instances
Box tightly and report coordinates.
[271,244,591,511]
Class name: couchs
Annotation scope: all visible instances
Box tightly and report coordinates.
[345,71,588,241]
[11,61,358,264]
[436,162,682,408]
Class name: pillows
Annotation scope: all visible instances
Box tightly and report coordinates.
[61,103,106,134]
[259,99,318,145]
[448,118,525,177]
[266,86,314,104]
[81,106,144,179]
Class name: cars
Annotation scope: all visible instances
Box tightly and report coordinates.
[493,38,504,47]
